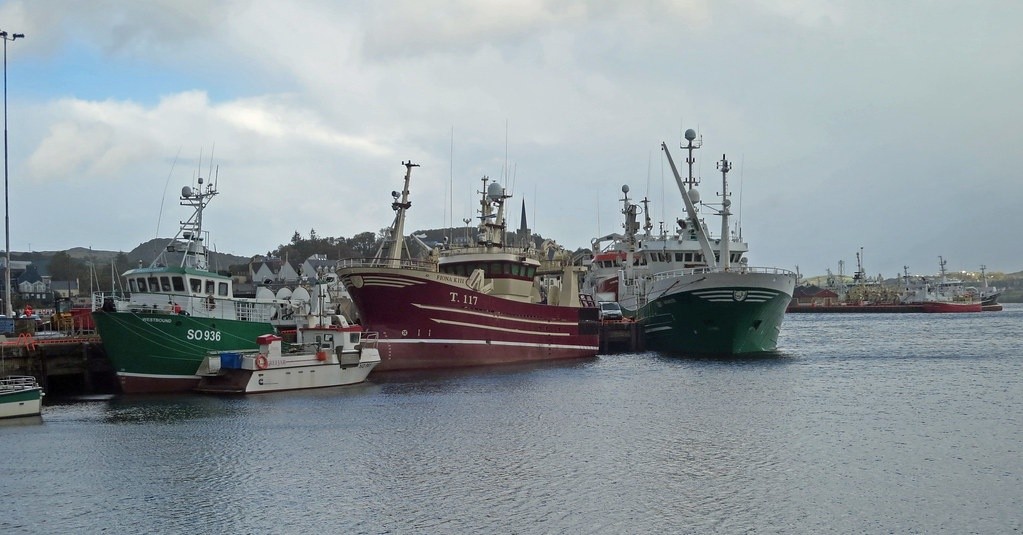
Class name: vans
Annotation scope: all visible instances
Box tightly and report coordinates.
[598,302,623,321]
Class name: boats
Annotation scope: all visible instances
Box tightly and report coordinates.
[586,126,796,360]
[86,140,384,394]
[0,374,44,419]
[338,121,600,376]
[806,244,1006,313]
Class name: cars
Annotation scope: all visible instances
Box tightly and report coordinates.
[3,295,90,339]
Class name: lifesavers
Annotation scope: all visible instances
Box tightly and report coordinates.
[256,354,269,369]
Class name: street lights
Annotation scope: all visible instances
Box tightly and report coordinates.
[0,31,25,319]
